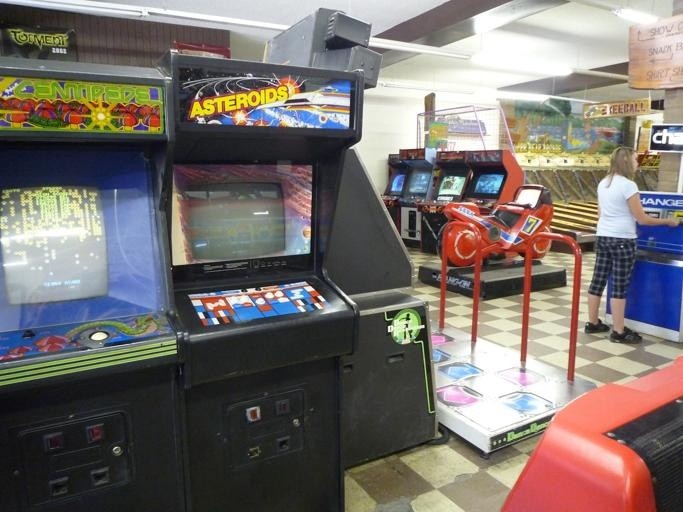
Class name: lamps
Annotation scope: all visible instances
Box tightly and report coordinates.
[0,0,472,62]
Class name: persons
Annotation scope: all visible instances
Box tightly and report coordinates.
[583,147,681,343]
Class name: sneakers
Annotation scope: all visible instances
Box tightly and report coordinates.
[585,319,610,333]
[610,327,642,344]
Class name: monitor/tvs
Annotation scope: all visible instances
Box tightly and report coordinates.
[169,162,316,279]
[437,175,466,199]
[515,188,542,209]
[1,177,112,310]
[409,172,429,195]
[389,174,405,193]
[472,172,504,197]
